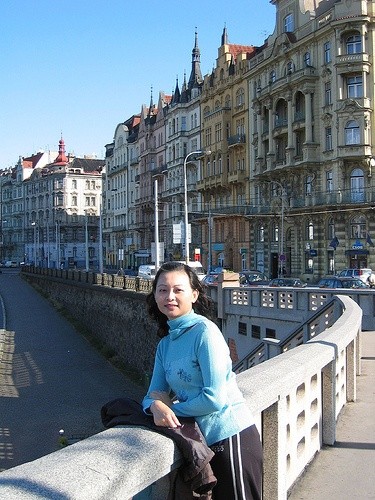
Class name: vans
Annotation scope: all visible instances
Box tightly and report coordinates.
[174,261,206,281]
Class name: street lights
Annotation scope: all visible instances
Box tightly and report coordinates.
[184,149,213,267]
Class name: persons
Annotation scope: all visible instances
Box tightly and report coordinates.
[141,261,265,500]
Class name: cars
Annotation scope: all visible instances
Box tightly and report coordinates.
[138,265,156,281]
[202,269,375,289]
